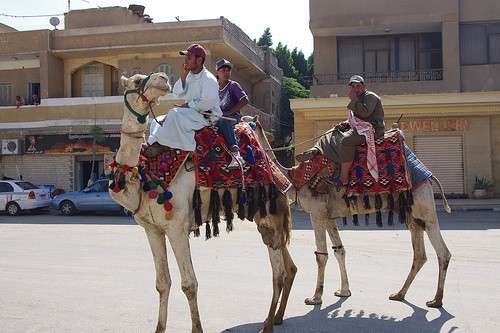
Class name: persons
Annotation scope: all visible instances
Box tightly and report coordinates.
[213,58,249,168]
[295,75,384,187]
[140,44,223,154]
[32,95,39,105]
[16,95,24,107]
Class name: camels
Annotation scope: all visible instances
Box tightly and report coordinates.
[108,71,298,333]
[240,114,451,308]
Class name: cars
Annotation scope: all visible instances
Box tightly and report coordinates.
[0,180,54,215]
[50,178,133,217]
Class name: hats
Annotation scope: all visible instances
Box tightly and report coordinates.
[216,58,233,70]
[179,44,206,58]
[348,75,364,86]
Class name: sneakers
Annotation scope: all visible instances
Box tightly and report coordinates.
[228,156,246,170]
[295,151,315,162]
[325,179,349,188]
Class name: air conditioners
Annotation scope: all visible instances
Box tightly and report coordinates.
[2,139,24,155]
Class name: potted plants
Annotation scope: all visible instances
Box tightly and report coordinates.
[472,176,494,199]
